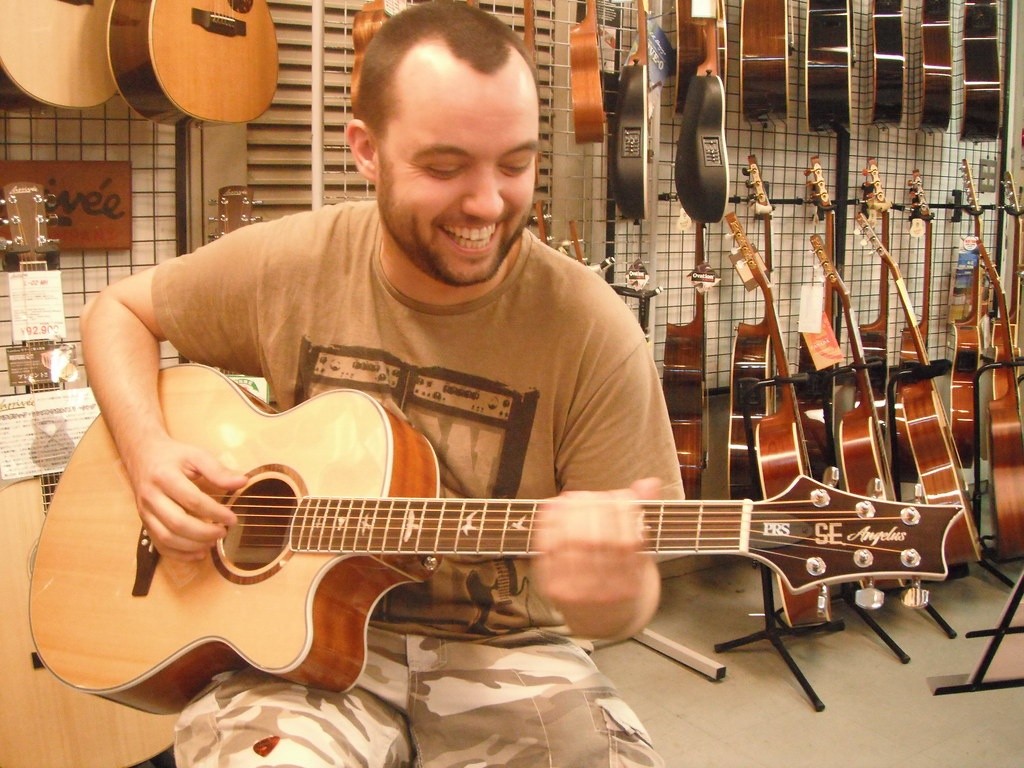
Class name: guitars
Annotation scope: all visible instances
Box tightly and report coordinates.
[202,183,265,249]
[651,153,1024,629]
[0,0,278,137]
[1,178,181,768]
[567,0,1008,225]
[27,363,968,721]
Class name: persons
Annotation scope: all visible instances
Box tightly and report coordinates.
[82,0,686,768]
